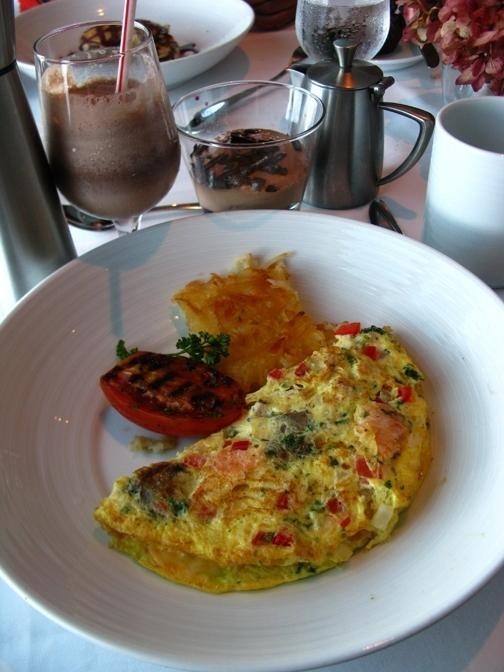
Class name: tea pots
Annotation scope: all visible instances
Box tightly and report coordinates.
[283,36,437,213]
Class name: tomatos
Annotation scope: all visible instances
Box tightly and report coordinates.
[99,352,248,438]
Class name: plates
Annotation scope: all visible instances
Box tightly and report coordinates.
[300,40,429,78]
[12,0,257,94]
[1,204,503,670]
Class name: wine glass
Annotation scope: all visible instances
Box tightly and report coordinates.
[29,16,182,238]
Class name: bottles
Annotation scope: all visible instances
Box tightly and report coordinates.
[1,1,79,311]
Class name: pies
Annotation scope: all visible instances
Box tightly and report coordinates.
[94,324,432,594]
[172,251,329,397]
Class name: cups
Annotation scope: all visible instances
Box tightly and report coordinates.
[170,77,327,211]
[420,96,503,292]
[293,0,394,62]
